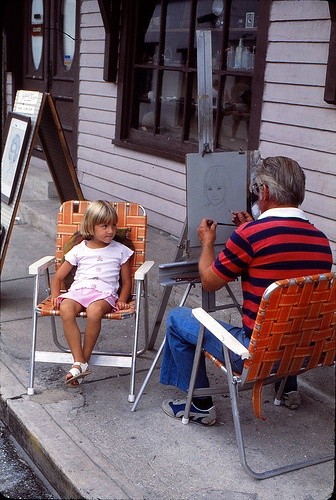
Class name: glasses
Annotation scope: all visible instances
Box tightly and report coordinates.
[252,183,269,195]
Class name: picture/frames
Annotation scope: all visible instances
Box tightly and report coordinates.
[0,110,32,204]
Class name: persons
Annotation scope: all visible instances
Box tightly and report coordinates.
[50,200,135,388]
[158,156,333,428]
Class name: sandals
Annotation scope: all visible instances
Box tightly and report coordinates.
[64,372,86,387]
[66,361,91,384]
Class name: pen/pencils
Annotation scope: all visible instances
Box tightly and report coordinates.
[228,209,252,221]
[171,277,196,282]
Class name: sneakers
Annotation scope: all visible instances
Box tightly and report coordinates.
[162,395,217,427]
[273,390,300,410]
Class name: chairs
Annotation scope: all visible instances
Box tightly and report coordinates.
[181,271,336,480]
[27,201,154,403]
